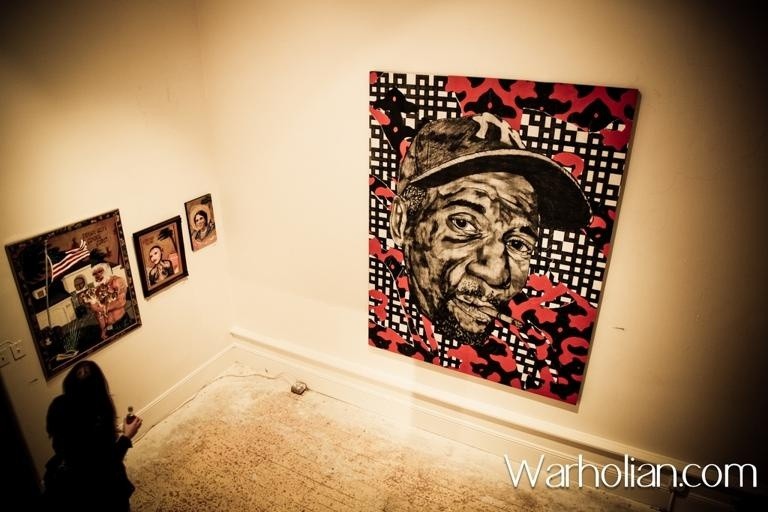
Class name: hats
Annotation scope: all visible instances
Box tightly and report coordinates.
[394,111,594,232]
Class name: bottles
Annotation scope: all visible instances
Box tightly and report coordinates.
[126,407,136,425]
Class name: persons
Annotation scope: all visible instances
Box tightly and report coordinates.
[388,112,592,345]
[42,394,133,512]
[62,361,144,497]
[193,210,214,242]
[147,245,176,287]
[91,262,131,336]
[81,288,108,340]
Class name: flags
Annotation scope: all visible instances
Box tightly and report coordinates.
[47,237,91,284]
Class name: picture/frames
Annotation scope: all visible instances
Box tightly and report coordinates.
[3,194,216,382]
